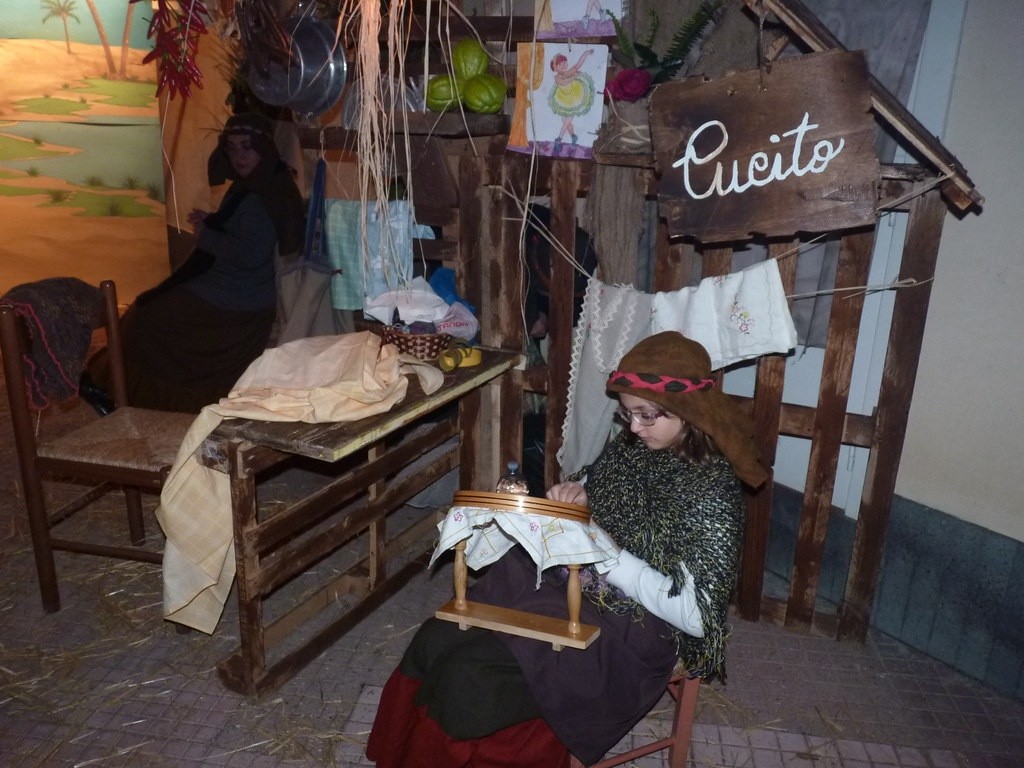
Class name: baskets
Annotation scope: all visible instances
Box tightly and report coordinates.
[353,308,453,362]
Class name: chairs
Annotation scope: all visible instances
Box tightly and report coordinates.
[0,279,200,634]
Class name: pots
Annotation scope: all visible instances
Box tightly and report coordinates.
[246,1,346,126]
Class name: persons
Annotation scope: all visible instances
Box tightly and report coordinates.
[78,113,306,421]
[364,329,749,768]
[524,202,597,340]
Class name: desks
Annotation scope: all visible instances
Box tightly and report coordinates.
[195,342,521,705]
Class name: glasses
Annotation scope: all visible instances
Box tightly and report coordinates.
[616,406,668,426]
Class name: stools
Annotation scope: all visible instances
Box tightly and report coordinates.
[568,665,702,768]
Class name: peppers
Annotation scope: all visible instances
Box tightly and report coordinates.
[142,0,209,100]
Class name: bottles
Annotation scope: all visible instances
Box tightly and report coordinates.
[496,461,530,496]
